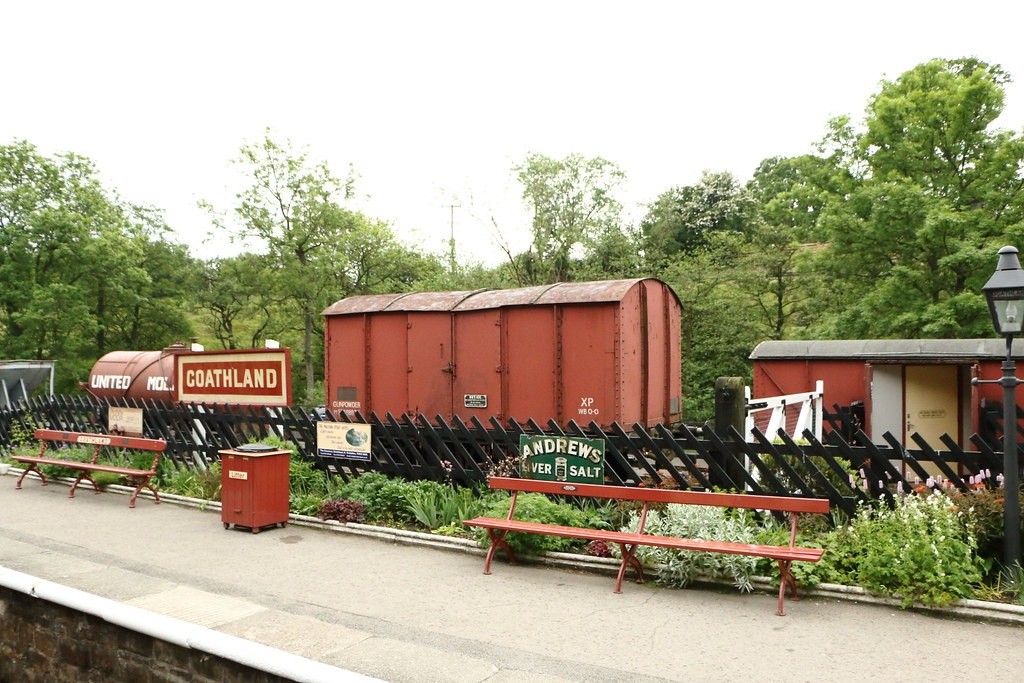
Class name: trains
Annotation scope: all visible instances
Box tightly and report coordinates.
[75,277,1023,519]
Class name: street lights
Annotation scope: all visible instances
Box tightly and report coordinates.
[970,245,1024,577]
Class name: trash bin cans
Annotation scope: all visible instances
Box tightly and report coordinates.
[216,443,294,534]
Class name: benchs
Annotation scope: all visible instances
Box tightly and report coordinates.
[9,429,166,508]
[461,477,828,615]
